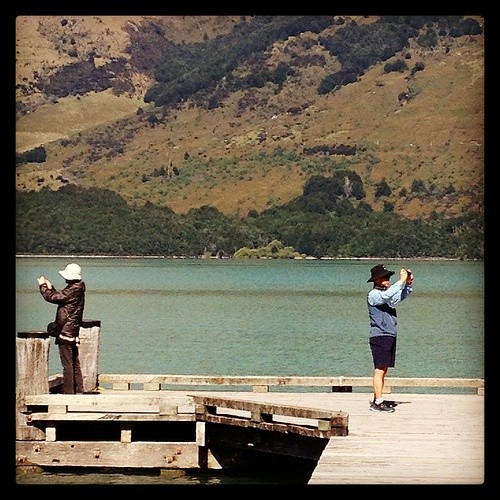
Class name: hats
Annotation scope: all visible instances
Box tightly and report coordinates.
[59,263,82,281]
[366,264,395,282]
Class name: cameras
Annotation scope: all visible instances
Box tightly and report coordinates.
[406,270,411,277]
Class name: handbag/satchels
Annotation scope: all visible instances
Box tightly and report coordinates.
[47,321,63,337]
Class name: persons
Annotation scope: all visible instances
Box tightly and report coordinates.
[364,262,414,414]
[36,262,89,396]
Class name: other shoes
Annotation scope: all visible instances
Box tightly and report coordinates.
[370,402,395,412]
[383,400,395,407]
